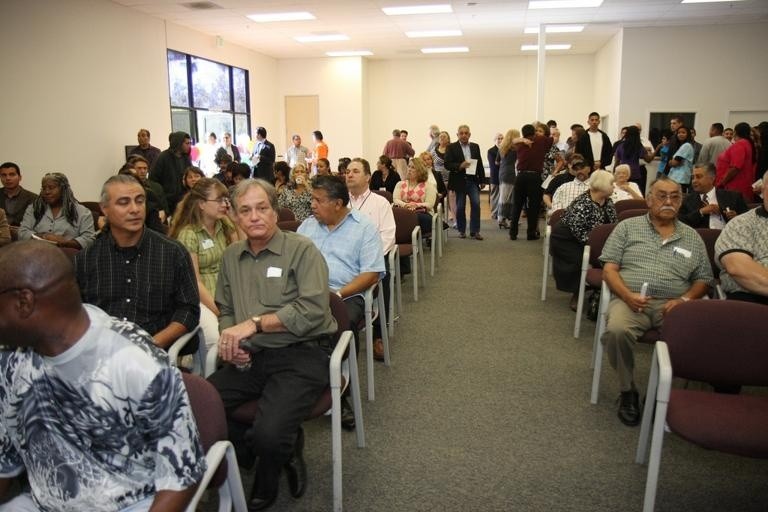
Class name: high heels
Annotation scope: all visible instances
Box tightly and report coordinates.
[499,223,511,229]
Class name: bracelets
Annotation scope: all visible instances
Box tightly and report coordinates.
[335,291,344,300]
[681,296,691,303]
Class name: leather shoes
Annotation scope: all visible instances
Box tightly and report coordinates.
[341,395,355,431]
[374,339,384,361]
[247,463,279,512]
[617,382,640,426]
[570,292,579,311]
[475,232,482,239]
[587,290,598,321]
[284,426,307,499]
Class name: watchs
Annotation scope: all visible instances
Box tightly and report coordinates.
[251,315,263,333]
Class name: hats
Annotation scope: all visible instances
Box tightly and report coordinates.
[569,154,584,169]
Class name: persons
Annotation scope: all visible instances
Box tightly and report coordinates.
[488,112,768,322]
[712,169,768,396]
[342,157,396,363]
[678,162,749,297]
[296,173,388,432]
[0,239,208,510]
[0,124,486,287]
[204,176,339,512]
[598,176,714,428]
[70,173,199,375]
[166,175,249,378]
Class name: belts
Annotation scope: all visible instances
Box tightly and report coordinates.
[518,170,538,174]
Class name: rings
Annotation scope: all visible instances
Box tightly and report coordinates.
[223,341,227,344]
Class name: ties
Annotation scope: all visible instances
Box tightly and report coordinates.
[701,194,708,207]
